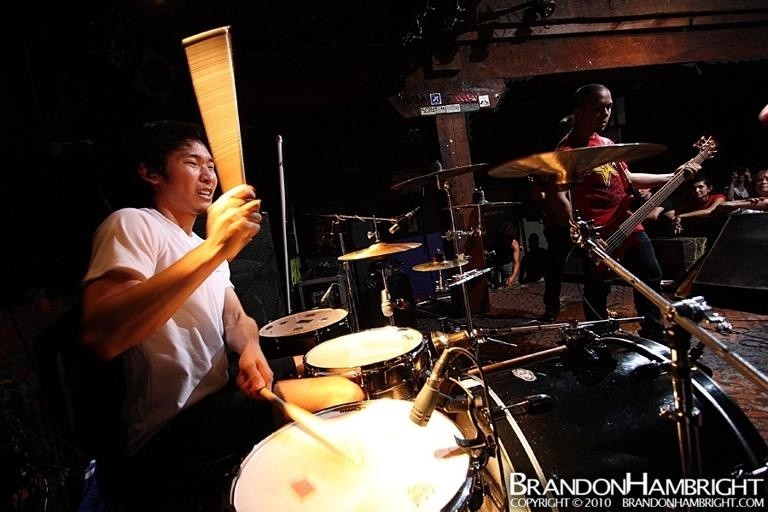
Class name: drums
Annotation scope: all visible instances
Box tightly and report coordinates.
[304,324,431,401]
[443,330,768,512]
[228,398,504,512]
[259,307,353,357]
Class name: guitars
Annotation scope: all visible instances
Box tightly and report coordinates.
[563,135,718,277]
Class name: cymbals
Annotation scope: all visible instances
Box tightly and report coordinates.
[337,242,422,261]
[411,259,468,273]
[391,162,487,189]
[487,142,636,178]
[445,200,522,211]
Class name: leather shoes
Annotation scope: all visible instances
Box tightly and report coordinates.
[544,311,556,321]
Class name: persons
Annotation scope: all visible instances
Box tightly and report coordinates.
[718,169,767,212]
[670,174,728,236]
[81,119,365,512]
[476,214,521,290]
[548,82,703,348]
[532,113,617,325]
[631,187,678,224]
[723,164,755,203]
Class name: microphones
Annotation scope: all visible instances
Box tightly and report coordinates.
[388,206,421,234]
[381,289,394,317]
[320,284,335,305]
[409,348,451,426]
